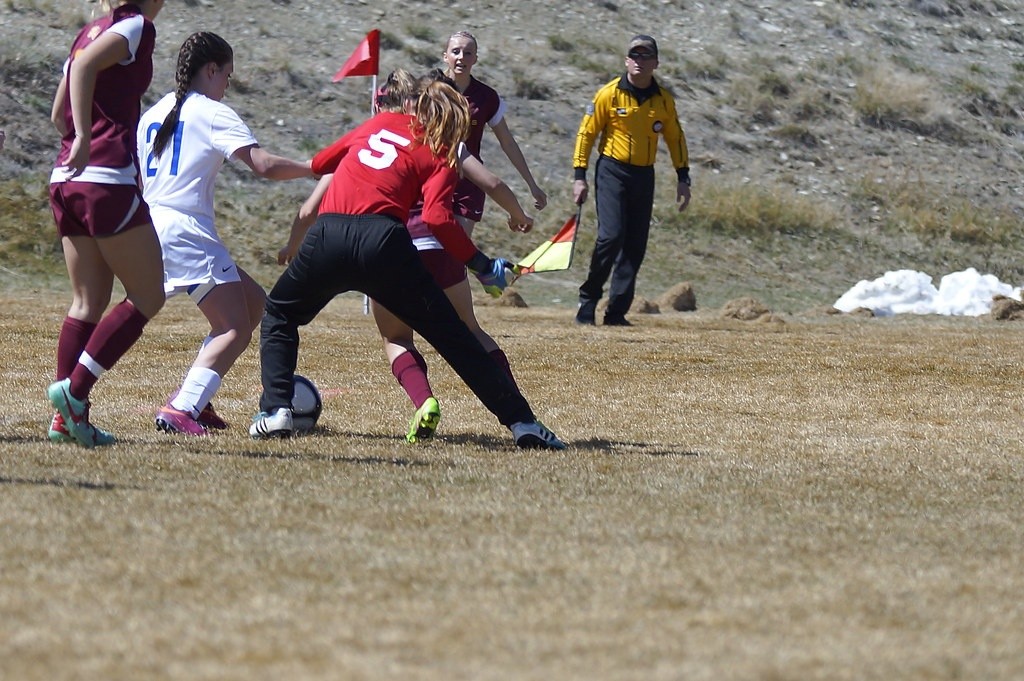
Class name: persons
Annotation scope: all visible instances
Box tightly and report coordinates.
[279,69,532,442]
[137,33,319,436]
[46,0,167,448]
[572,36,690,326]
[246,68,568,449]
[440,30,548,239]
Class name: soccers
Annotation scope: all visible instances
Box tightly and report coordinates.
[258,373,323,430]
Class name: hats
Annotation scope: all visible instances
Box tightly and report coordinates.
[628,34,658,59]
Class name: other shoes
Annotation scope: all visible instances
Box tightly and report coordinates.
[577,303,596,325]
[603,316,633,326]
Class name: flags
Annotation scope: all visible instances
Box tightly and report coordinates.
[332,29,380,82]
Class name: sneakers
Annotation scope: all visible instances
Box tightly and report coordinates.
[48,407,115,448]
[155,402,206,436]
[510,419,565,449]
[408,396,441,443]
[249,407,293,437]
[48,377,95,449]
[198,402,226,429]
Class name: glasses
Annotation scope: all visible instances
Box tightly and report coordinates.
[628,51,652,61]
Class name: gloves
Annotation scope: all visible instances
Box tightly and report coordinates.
[477,258,521,296]
